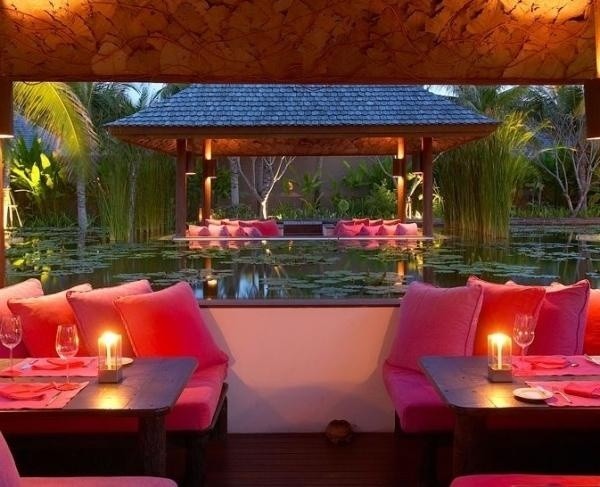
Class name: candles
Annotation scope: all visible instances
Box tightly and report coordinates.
[106,340,113,370]
[497,340,503,370]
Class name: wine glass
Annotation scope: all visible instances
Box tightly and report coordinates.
[55,324,80,388]
[513,312,534,367]
[1,315,23,375]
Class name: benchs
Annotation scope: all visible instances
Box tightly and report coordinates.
[19,475,177,487]
[450,469,600,487]
[0,362,231,486]
[375,363,600,487]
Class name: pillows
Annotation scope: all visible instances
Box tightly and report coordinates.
[335,218,418,243]
[0,277,230,375]
[187,218,281,248]
[385,275,598,374]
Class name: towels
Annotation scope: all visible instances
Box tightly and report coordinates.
[1,383,56,400]
[563,381,600,398]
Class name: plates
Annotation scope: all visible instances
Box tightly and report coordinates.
[513,388,554,401]
[106,359,135,368]
